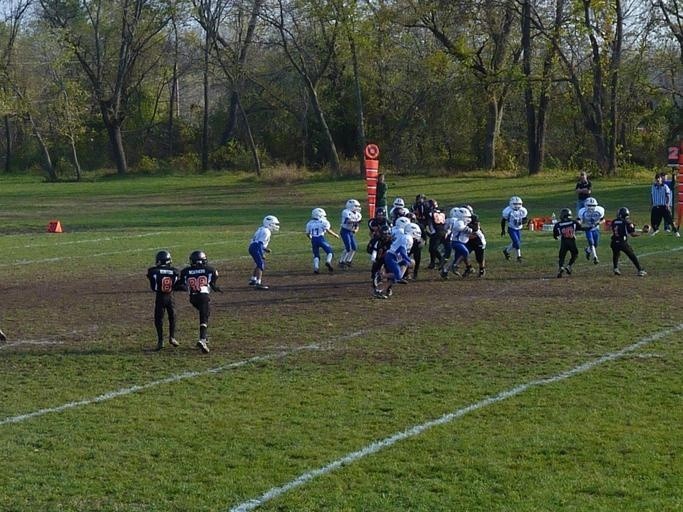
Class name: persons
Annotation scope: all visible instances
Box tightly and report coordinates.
[339,198,362,267]
[179,250,220,354]
[573,171,591,223]
[247,215,280,289]
[552,207,580,278]
[146,251,180,351]
[367,195,486,300]
[376,172,387,218]
[658,172,673,230]
[648,174,680,238]
[304,208,340,273]
[577,197,605,265]
[499,195,528,262]
[608,206,646,277]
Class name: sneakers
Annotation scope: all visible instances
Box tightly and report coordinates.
[313,266,321,274]
[636,270,647,276]
[197,339,210,355]
[502,248,511,260]
[613,268,622,275]
[424,257,476,281]
[338,260,352,272]
[478,266,486,278]
[156,337,165,351]
[517,256,523,263]
[371,273,417,300]
[584,248,590,260]
[248,278,270,291]
[593,256,599,264]
[556,266,573,278]
[325,261,333,273]
[650,227,682,239]
[168,337,179,346]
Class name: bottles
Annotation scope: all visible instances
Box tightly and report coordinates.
[529,220,535,232]
[552,211,557,225]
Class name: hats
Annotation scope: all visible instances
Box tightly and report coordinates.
[655,172,668,178]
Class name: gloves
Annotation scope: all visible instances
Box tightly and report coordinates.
[214,286,224,295]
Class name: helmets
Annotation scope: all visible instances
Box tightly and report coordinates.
[368,208,392,237]
[189,250,206,265]
[416,194,438,211]
[559,208,574,222]
[262,215,280,232]
[509,195,524,212]
[346,199,361,213]
[449,204,474,218]
[311,208,327,220]
[391,197,422,241]
[616,207,631,220]
[585,197,599,211]
[155,251,172,264]
